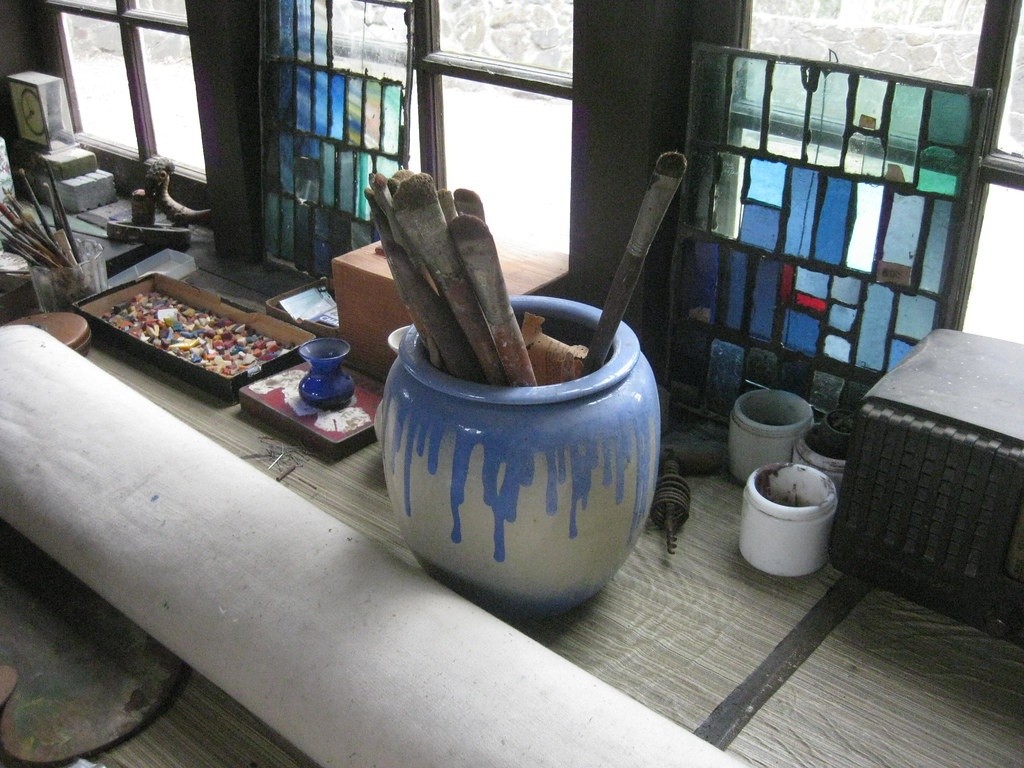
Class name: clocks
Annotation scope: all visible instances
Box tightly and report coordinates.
[7,71,48,148]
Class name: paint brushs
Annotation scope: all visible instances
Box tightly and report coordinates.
[364,171,540,386]
[579,151,688,375]
[0,158,82,267]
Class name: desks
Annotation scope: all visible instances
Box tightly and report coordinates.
[0,198,1024,768]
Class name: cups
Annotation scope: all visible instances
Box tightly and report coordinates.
[727,390,814,487]
[26,240,108,315]
[790,422,846,492]
[738,462,838,577]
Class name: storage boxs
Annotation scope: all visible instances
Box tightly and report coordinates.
[73,271,317,405]
[830,329,1024,650]
[266,276,339,339]
[331,239,441,382]
